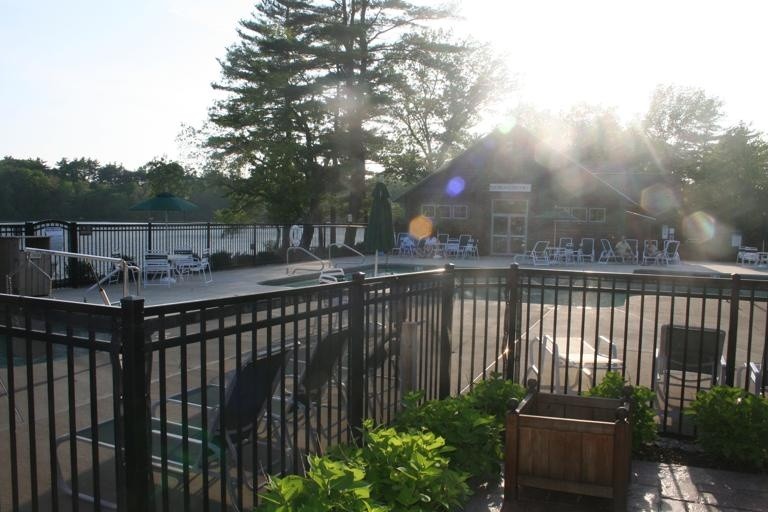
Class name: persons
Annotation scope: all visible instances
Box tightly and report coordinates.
[646,239,661,257]
[614,235,635,265]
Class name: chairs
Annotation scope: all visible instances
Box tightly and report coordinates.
[390,233,479,261]
[524,323,728,437]
[514,238,682,267]
[48,341,303,512]
[108,249,212,290]
[152,317,428,491]
[736,247,768,268]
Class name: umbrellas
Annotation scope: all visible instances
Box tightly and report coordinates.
[362,181,398,277]
[127,191,199,253]
[532,209,578,246]
